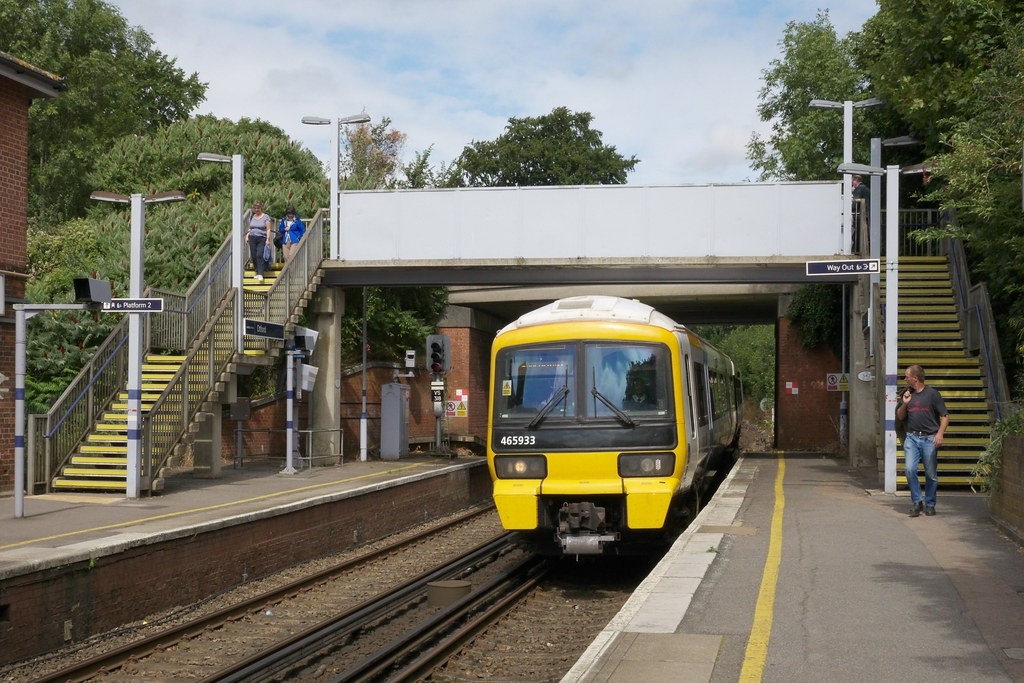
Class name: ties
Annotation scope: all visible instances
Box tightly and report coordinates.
[638,398,641,403]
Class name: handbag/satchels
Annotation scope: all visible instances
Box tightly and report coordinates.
[264,244,271,261]
[274,235,282,249]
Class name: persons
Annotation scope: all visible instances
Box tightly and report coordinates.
[853,175,870,253]
[245,199,271,281]
[893,365,950,518]
[279,206,306,276]
[627,378,648,406]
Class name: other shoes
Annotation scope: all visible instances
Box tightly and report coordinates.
[910,501,923,517]
[254,274,263,281]
[926,506,935,516]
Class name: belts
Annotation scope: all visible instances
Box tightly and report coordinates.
[910,429,938,435]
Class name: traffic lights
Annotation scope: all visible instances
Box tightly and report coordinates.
[425,334,450,373]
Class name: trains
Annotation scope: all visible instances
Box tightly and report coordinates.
[484,293,742,576]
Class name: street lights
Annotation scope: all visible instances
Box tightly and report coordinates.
[302,113,373,261]
[196,151,245,355]
[88,189,187,499]
[866,134,927,357]
[807,95,889,257]
[835,158,944,494]
